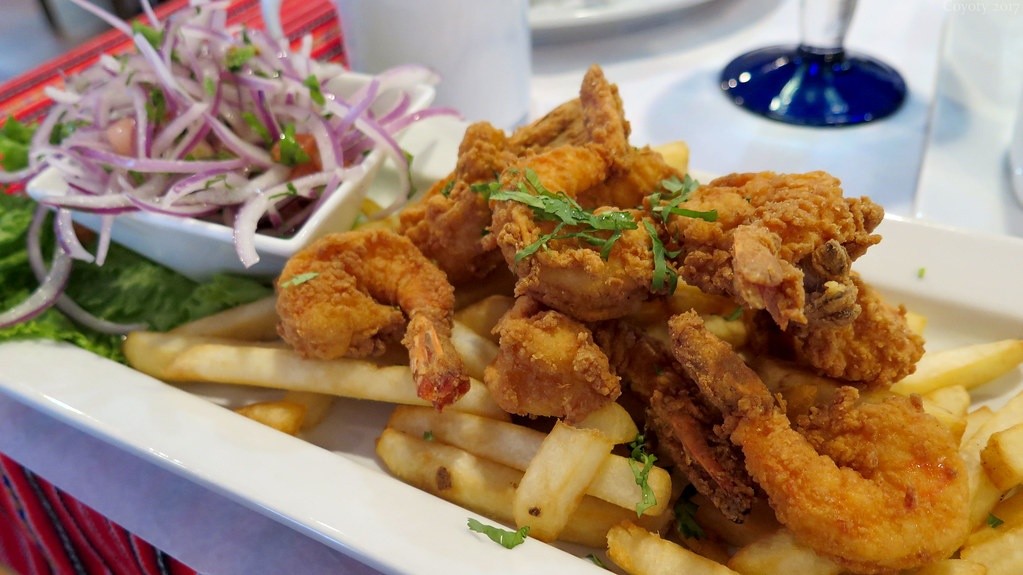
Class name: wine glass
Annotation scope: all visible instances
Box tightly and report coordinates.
[720,0,906,127]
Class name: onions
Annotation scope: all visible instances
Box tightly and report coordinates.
[1,0,455,333]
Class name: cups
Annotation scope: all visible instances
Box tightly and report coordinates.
[332,0,532,138]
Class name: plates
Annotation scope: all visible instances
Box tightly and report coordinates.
[531,1,712,41]
[0,106,1023,575]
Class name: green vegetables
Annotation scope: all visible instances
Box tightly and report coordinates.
[0,115,272,368]
[474,170,716,291]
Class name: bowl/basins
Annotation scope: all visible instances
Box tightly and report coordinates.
[25,73,436,284]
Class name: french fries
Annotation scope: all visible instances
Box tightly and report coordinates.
[122,297,1023,575]
